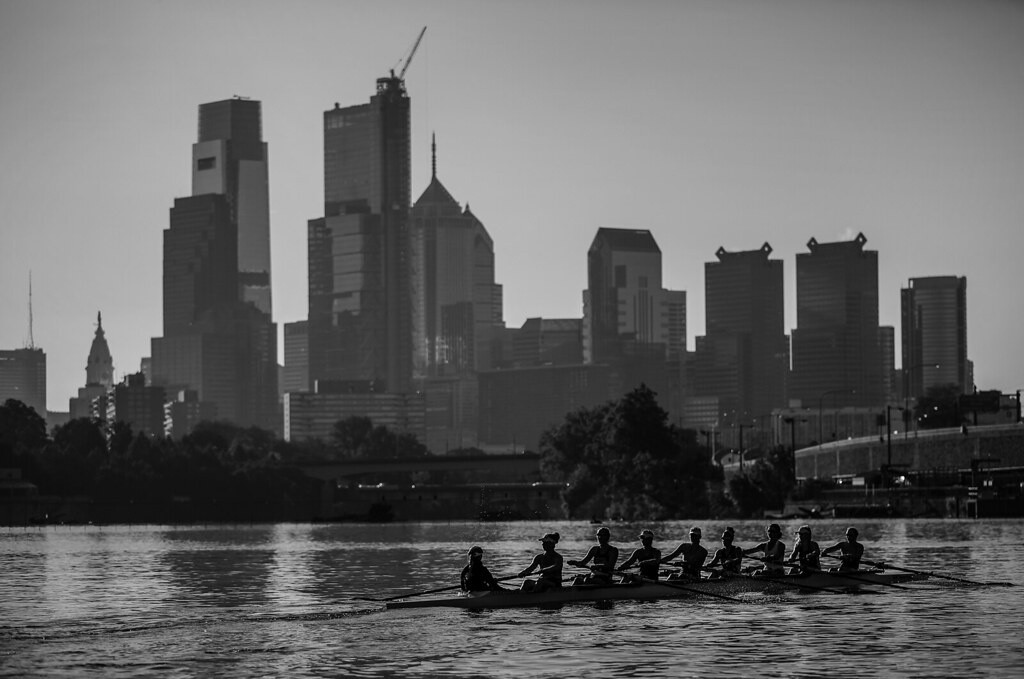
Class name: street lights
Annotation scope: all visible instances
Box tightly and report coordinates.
[903,361,940,439]
[783,415,795,480]
[738,424,752,472]
[707,419,717,466]
[818,388,856,443]
[886,404,902,466]
[796,419,808,424]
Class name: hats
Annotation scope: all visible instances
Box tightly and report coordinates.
[640,530,654,540]
[597,527,610,536]
[795,524,812,534]
[845,528,859,537]
[721,527,734,540]
[539,532,560,543]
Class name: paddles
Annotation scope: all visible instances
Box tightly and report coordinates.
[741,557,815,573]
[562,563,640,585]
[658,563,729,571]
[563,559,750,607]
[353,571,546,603]
[745,553,913,592]
[661,557,845,594]
[821,550,983,586]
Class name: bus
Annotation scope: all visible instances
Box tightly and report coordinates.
[978,467,1024,490]
[792,474,816,487]
[907,468,973,484]
[814,474,857,485]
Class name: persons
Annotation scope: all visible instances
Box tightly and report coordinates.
[742,523,785,575]
[618,529,661,583]
[573,528,618,586]
[461,546,505,592]
[660,526,708,580]
[518,531,563,591]
[789,525,821,575]
[822,527,864,572]
[707,526,742,578]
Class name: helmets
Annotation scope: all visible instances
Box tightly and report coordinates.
[689,527,701,536]
[469,545,483,556]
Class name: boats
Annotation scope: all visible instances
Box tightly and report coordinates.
[387,561,931,621]
